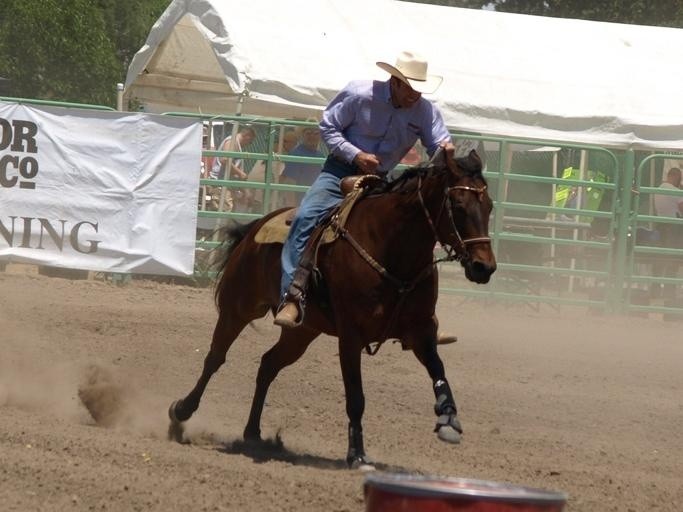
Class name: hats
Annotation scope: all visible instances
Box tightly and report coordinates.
[293,117,321,132]
[372,49,444,97]
[277,132,298,144]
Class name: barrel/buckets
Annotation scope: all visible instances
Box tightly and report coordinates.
[363,471,567,512]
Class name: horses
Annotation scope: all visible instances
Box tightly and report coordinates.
[168,146,500,474]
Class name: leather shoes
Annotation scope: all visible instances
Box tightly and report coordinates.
[400,328,459,351]
[274,302,298,324]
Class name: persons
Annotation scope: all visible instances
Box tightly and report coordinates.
[650,166,682,300]
[240,131,298,217]
[270,49,456,351]
[277,115,326,210]
[206,126,256,242]
